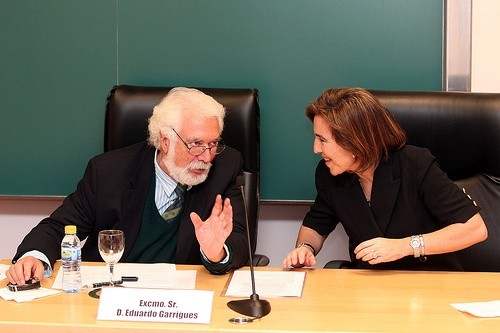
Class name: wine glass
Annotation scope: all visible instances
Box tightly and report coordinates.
[96,230,125,297]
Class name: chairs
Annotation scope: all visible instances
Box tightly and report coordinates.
[323,89,500,273]
[103,84,270,266]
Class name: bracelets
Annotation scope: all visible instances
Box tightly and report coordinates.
[295,242,316,257]
[419,234,425,256]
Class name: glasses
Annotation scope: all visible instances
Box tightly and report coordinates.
[169,125,227,156]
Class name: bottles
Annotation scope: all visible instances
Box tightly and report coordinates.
[61,225,83,294]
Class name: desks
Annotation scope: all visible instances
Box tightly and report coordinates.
[0,260,500,333]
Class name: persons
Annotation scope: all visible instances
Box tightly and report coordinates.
[5,87,258,286]
[283,88,488,272]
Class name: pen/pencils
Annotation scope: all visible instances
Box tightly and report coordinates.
[82,279,123,289]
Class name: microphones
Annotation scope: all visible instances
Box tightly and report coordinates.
[227,176,271,318]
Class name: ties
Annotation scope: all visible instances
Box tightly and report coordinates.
[161,182,188,223]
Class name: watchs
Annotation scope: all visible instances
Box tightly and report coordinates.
[409,235,421,257]
[7,277,41,292]
